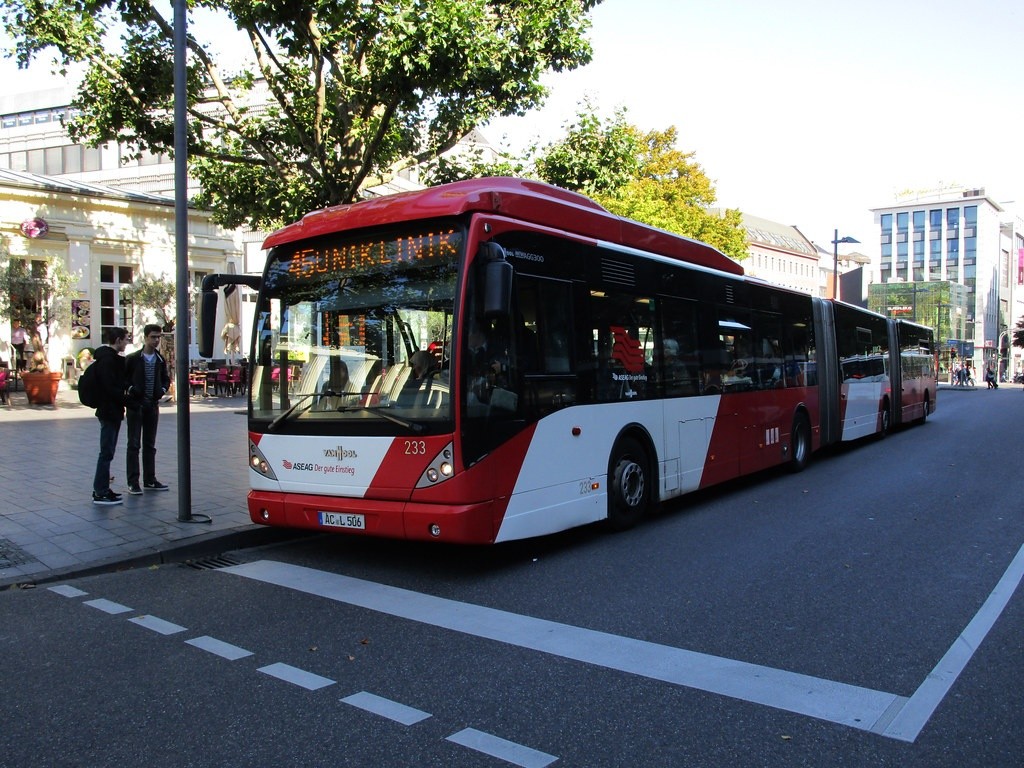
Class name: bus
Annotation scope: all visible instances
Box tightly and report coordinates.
[841,353,935,382]
[195,176,937,545]
[773,362,817,387]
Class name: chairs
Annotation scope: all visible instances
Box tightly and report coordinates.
[190,359,302,397]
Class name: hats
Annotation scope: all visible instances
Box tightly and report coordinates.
[663,339,678,358]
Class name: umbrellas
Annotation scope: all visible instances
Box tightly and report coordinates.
[222,261,241,365]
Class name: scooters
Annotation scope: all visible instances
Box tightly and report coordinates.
[1014,372,1023,383]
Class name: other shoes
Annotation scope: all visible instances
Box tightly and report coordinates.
[16,373,21,380]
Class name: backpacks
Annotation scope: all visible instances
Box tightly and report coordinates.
[78,358,105,409]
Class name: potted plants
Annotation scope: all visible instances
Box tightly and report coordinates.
[0,250,82,405]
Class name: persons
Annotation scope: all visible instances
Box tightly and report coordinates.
[0,356,10,405]
[466,323,510,378]
[84,327,131,505]
[10,319,27,368]
[955,364,998,390]
[318,359,360,409]
[124,324,171,494]
[426,330,450,363]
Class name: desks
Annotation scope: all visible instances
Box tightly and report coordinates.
[207,370,219,395]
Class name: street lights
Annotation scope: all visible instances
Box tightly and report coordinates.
[1001,324,1009,382]
[937,305,953,385]
[913,289,930,322]
[961,319,973,387]
[831,237,861,299]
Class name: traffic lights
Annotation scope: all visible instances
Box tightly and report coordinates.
[951,348,956,358]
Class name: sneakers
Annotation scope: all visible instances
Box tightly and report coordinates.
[92,490,122,500]
[128,483,143,494]
[144,481,168,490]
[94,494,123,504]
